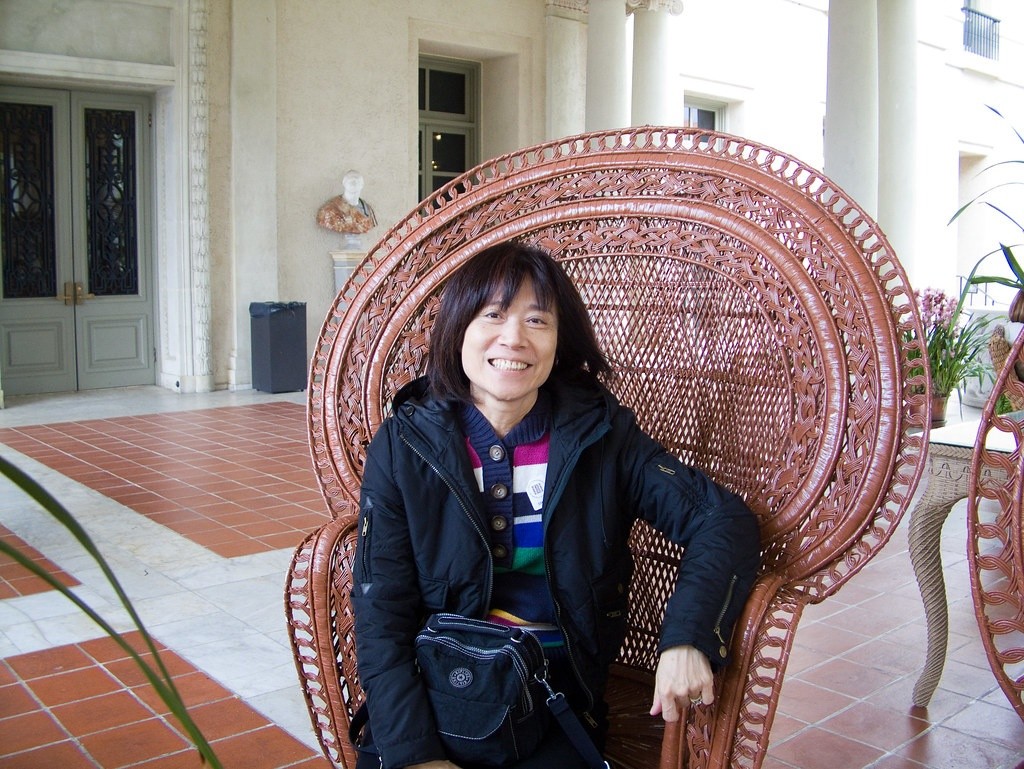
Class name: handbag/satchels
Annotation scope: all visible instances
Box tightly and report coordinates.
[412,612,551,766]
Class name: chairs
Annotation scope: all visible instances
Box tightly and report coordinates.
[968,327,1023,724]
[283,123,932,768]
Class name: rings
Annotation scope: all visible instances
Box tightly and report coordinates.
[690,695,702,706]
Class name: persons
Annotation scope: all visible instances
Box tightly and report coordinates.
[318,170,378,234]
[349,242,760,769]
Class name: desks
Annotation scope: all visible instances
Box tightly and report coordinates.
[897,410,1024,709]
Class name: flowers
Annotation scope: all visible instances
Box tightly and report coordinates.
[895,285,979,391]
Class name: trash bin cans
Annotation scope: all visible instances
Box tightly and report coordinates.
[249,301,308,392]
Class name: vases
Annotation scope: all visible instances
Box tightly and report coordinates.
[900,385,954,429]
